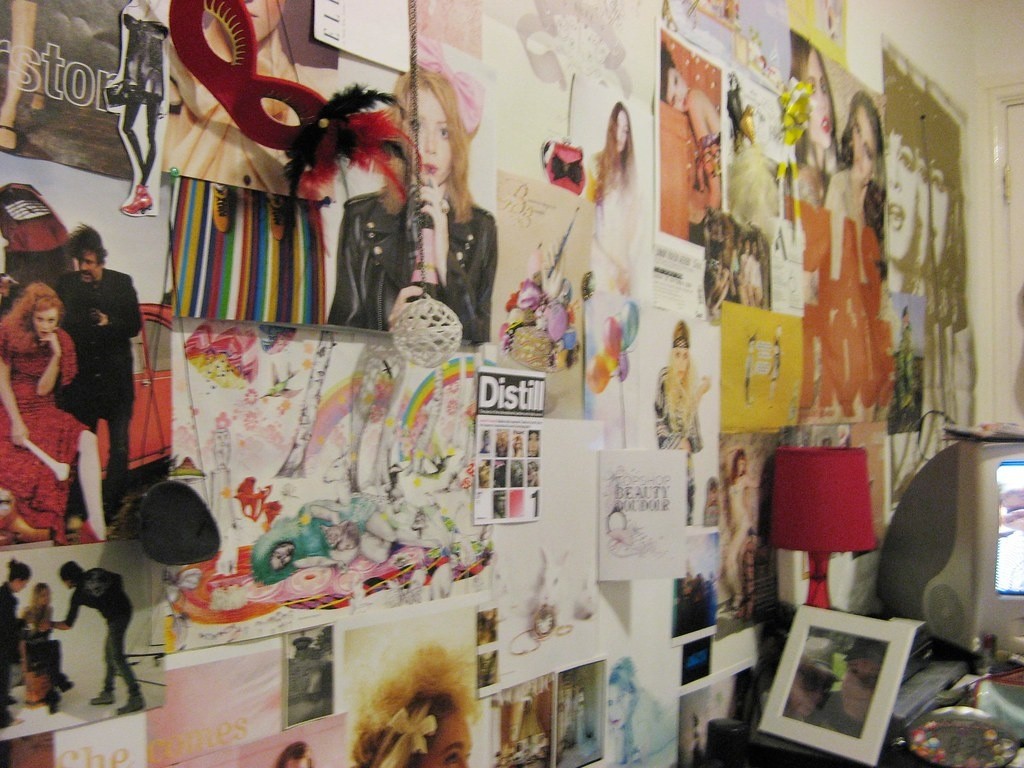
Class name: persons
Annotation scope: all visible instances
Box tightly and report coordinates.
[0,183,142,545]
[164,0,976,718]
[782,633,889,738]
[0,558,146,727]
[0,0,55,153]
[275,741,315,768]
[610,656,673,761]
[352,659,472,768]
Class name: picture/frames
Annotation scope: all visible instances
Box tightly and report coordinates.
[756,605,918,767]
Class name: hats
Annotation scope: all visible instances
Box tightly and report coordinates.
[843,638,886,662]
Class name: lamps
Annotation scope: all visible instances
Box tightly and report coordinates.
[769,446,878,608]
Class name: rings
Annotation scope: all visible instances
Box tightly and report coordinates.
[440,199,449,214]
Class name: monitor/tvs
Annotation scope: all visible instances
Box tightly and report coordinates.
[876,440,1024,667]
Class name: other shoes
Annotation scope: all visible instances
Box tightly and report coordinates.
[118,695,145,716]
[91,690,113,706]
[79,521,112,544]
[55,676,74,692]
[0,507,50,545]
[45,692,62,714]
